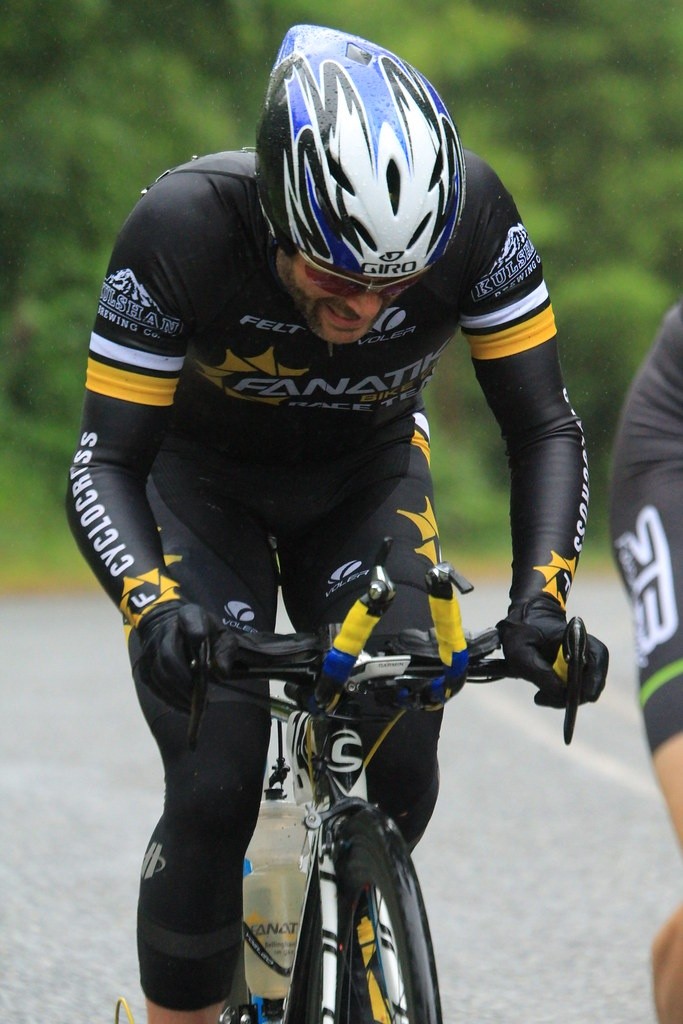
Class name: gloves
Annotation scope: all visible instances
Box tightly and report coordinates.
[494,595,608,711]
[136,599,219,719]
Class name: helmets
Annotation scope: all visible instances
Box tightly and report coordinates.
[256,23,466,279]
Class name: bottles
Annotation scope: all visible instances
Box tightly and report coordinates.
[242,788,314,999]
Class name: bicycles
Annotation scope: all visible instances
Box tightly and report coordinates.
[115,536,585,1024]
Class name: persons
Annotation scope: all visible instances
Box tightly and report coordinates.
[608,302,683,1024]
[64,24,611,1024]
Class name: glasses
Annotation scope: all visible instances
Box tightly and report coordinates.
[294,249,442,307]
